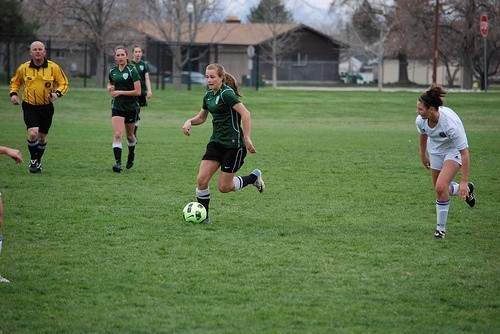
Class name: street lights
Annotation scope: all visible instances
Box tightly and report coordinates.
[186,2,195,90]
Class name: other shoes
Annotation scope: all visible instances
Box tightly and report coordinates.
[113,165,123,173]
[126,153,135,170]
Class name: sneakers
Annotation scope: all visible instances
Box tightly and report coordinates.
[251,169,266,194]
[204,217,210,223]
[465,182,476,208]
[29,159,38,172]
[433,229,446,239]
[37,163,42,171]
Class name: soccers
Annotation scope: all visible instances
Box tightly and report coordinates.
[181,201,208,225]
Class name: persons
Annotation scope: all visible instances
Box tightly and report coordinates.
[415,86,476,239]
[10,41,68,173]
[0,145,25,283]
[107,47,142,172]
[182,64,265,223]
[130,46,152,144]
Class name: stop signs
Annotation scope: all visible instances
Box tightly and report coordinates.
[480,15,489,36]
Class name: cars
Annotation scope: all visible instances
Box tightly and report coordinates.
[150,64,205,85]
